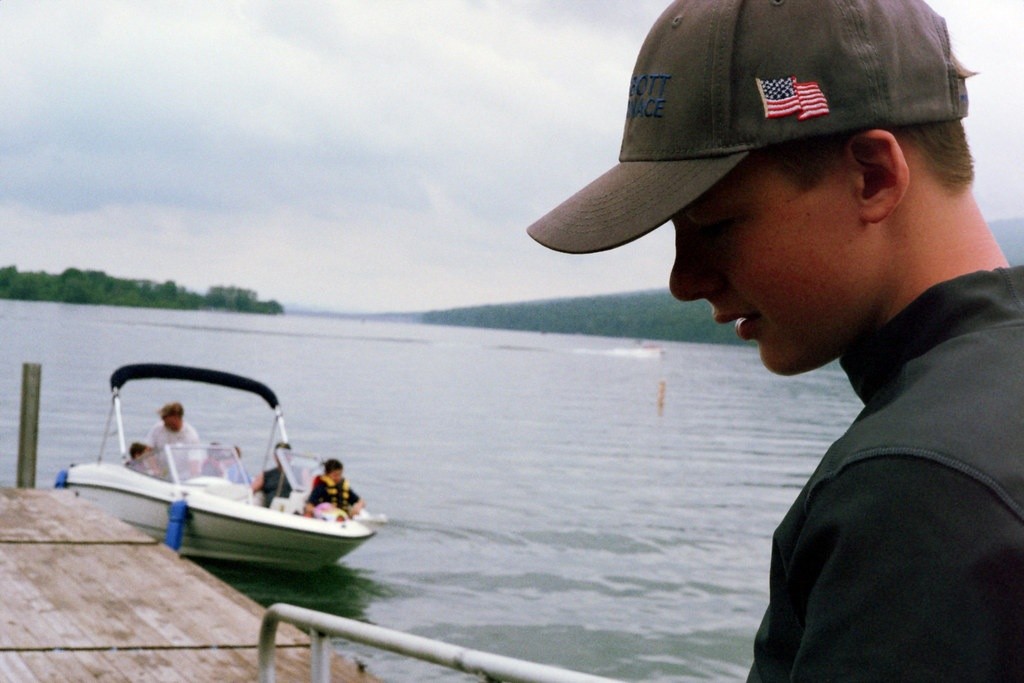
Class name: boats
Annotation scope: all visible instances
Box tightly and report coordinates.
[54,362,375,576]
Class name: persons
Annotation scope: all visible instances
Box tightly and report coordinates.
[526,1,1024,683]
[129,403,364,528]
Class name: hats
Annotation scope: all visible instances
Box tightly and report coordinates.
[526,1,977,257]
